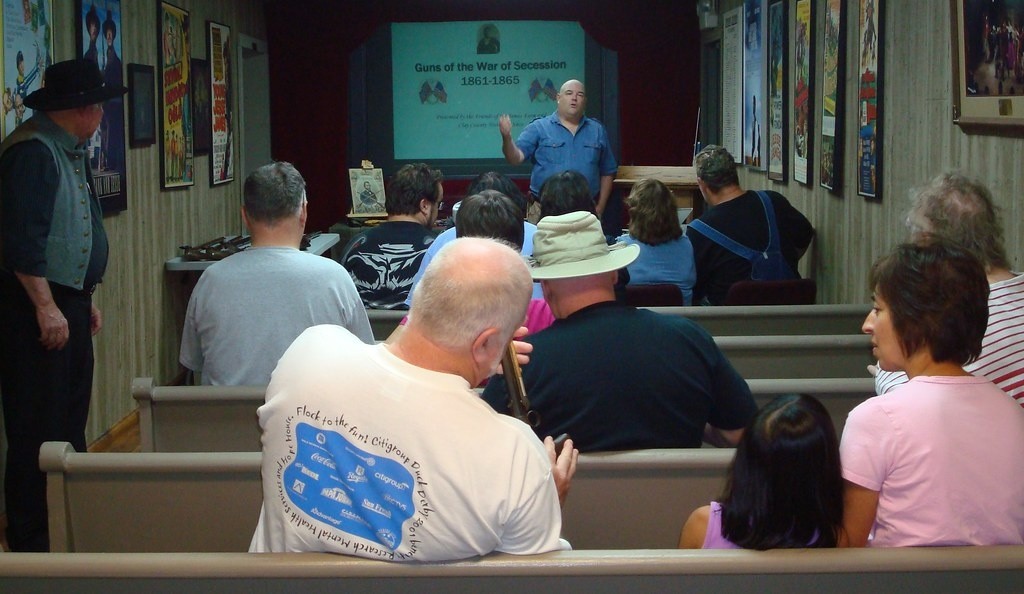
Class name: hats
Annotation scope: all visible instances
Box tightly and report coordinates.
[23,60,122,111]
[103,11,116,39]
[523,211,640,282]
[86,6,100,36]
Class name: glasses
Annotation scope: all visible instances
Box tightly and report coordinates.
[435,200,445,211]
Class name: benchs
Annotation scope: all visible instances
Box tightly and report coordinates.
[0,304,1024,594]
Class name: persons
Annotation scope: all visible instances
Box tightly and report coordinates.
[341,161,444,311]
[0,59,129,553]
[85,5,122,86]
[403,171,544,307]
[499,79,617,225]
[179,161,374,387]
[838,239,1024,547]
[869,171,1024,408]
[248,237,578,562]
[480,210,758,452]
[386,189,557,384]
[614,179,695,306]
[536,169,631,287]
[678,393,844,550]
[685,144,813,305]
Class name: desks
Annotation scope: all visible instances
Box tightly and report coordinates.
[165,233,339,386]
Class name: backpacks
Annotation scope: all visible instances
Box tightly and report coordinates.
[687,190,794,281]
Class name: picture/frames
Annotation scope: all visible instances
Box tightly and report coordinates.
[949,0,1024,127]
